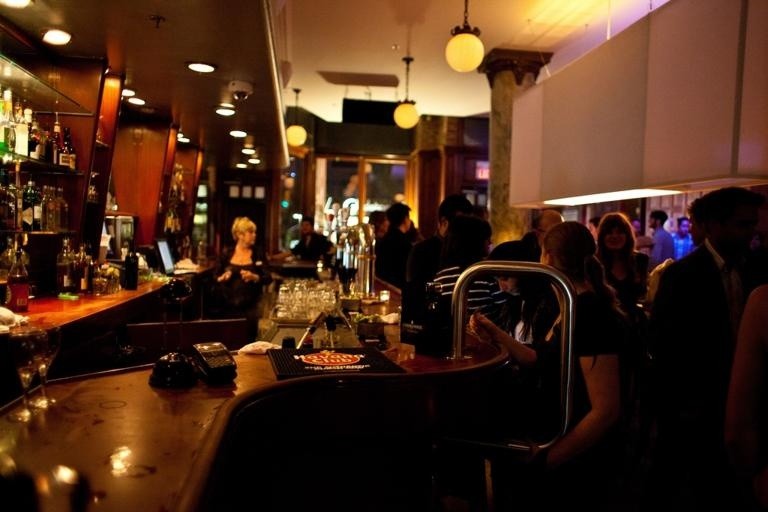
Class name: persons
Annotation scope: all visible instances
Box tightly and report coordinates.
[206,216,273,317]
[292,217,337,261]
[370,186,768,512]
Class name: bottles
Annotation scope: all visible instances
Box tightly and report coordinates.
[23,170,33,231]
[23,108,40,159]
[57,235,74,290]
[58,145,70,168]
[48,138,58,165]
[4,233,30,311]
[33,178,42,231]
[53,112,64,147]
[57,185,68,230]
[0,90,16,152]
[47,184,61,230]
[64,128,76,170]
[9,171,23,231]
[67,232,74,253]
[40,185,49,231]
[14,96,28,156]
[32,111,46,160]
[0,169,16,228]
[124,237,138,290]
[76,239,89,292]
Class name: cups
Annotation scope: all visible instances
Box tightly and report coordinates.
[380,290,390,304]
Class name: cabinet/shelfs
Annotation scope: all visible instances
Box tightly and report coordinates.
[0,23,108,306]
[113,124,177,253]
[77,65,125,274]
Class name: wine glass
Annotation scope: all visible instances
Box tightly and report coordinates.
[338,208,351,228]
[323,209,332,232]
[273,279,338,322]
[9,327,48,421]
[36,326,63,402]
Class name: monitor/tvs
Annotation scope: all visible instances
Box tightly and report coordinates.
[155,237,179,275]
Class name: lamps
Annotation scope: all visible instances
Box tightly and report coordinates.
[445,0,485,73]
[286,88,307,146]
[393,56,420,129]
